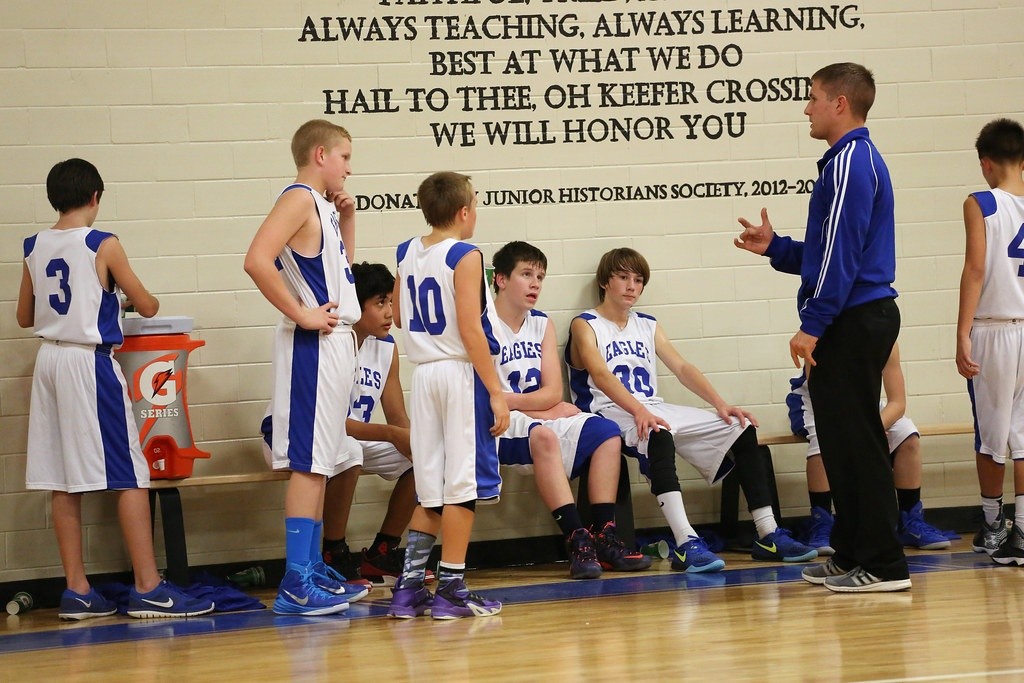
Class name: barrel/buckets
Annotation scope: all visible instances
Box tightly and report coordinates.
[118,314,210,479]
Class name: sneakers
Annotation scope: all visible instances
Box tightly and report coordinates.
[671,535,725,572]
[808,506,835,556]
[432,578,502,619]
[361,547,406,580]
[973,512,1008,556]
[322,543,360,579]
[896,501,950,549]
[752,527,818,561]
[310,561,368,603]
[824,565,912,592]
[273,569,350,616]
[565,528,601,579]
[386,575,434,618]
[58,587,117,619]
[802,558,850,584]
[127,579,215,618]
[594,521,651,570]
[992,524,1024,565]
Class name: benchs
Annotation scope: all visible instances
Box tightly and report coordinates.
[141,422,979,588]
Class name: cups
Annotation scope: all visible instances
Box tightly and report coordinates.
[639,540,671,561]
[6,592,34,616]
[226,566,265,589]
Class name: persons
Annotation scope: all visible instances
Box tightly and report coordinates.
[492,242,649,578]
[244,119,367,614]
[955,119,1024,566]
[563,248,818,573]
[261,262,434,592]
[17,159,215,618]
[734,63,912,591]
[786,339,951,551]
[385,171,511,620]
